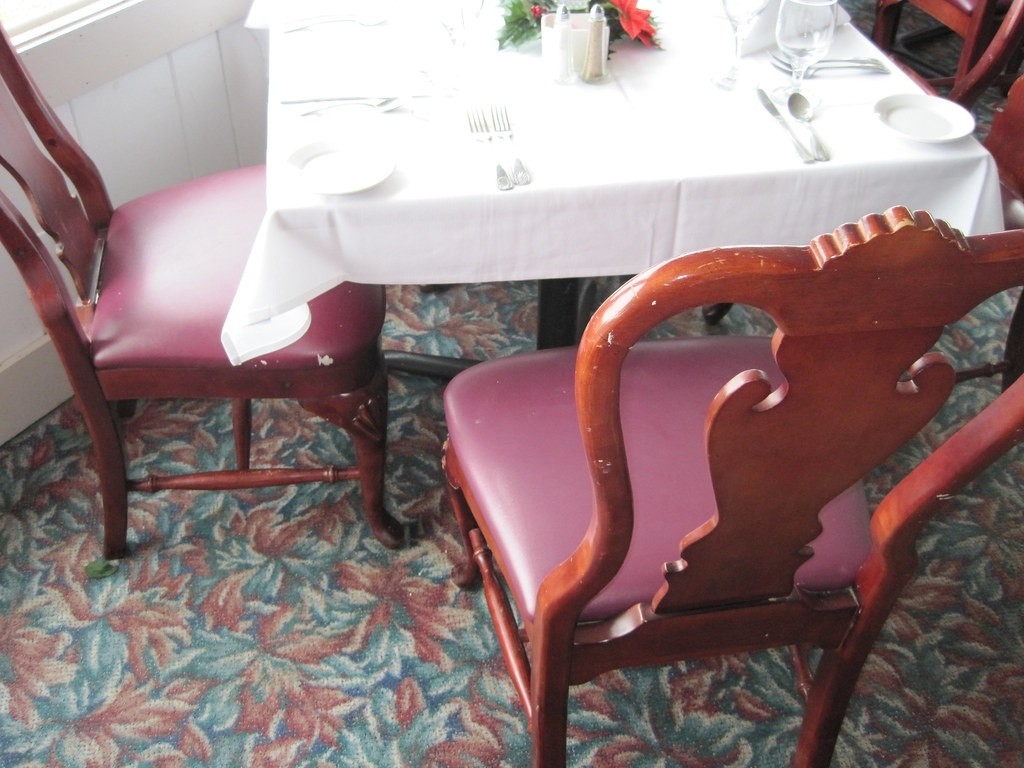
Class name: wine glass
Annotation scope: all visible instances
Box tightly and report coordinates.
[772,0,838,113]
[713,0,774,89]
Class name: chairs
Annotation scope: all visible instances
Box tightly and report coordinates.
[438,207,1024,768]
[0,22,406,561]
[872,0,1024,236]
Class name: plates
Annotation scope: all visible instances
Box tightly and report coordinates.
[873,92,975,143]
[289,140,396,198]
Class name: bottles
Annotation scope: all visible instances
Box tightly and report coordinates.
[580,4,607,81]
[550,4,576,84]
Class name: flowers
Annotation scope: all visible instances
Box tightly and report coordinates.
[497,1,660,51]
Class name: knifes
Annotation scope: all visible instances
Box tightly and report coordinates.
[756,89,814,163]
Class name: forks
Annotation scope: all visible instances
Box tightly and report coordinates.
[774,64,889,78]
[467,106,511,189]
[490,103,531,186]
[771,54,884,71]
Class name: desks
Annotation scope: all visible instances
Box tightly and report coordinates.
[219,0,1007,381]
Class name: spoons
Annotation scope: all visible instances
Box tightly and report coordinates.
[300,97,407,115]
[787,92,831,163]
